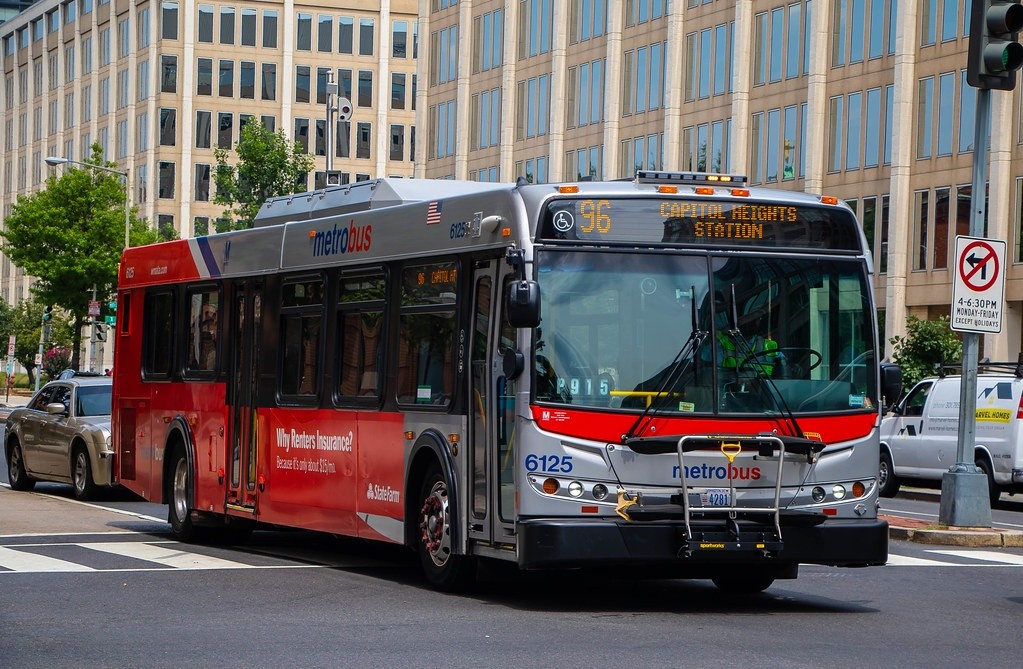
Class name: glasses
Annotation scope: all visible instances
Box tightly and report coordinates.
[744,315,762,321]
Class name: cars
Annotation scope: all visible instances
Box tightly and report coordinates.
[3,376,113,502]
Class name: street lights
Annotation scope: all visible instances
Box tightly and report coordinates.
[45,158,131,248]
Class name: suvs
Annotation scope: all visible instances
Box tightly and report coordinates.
[55,369,103,380]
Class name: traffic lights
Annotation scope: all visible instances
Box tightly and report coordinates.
[43,306,53,321]
[966,0,1023,91]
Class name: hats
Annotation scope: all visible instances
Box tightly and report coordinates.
[736,299,766,318]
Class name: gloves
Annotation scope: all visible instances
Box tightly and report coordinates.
[791,364,803,377]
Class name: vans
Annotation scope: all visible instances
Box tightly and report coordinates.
[880,361,1023,509]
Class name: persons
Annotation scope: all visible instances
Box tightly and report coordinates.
[104,368,113,376]
[701,303,804,392]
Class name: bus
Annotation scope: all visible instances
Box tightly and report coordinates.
[110,167,890,594]
